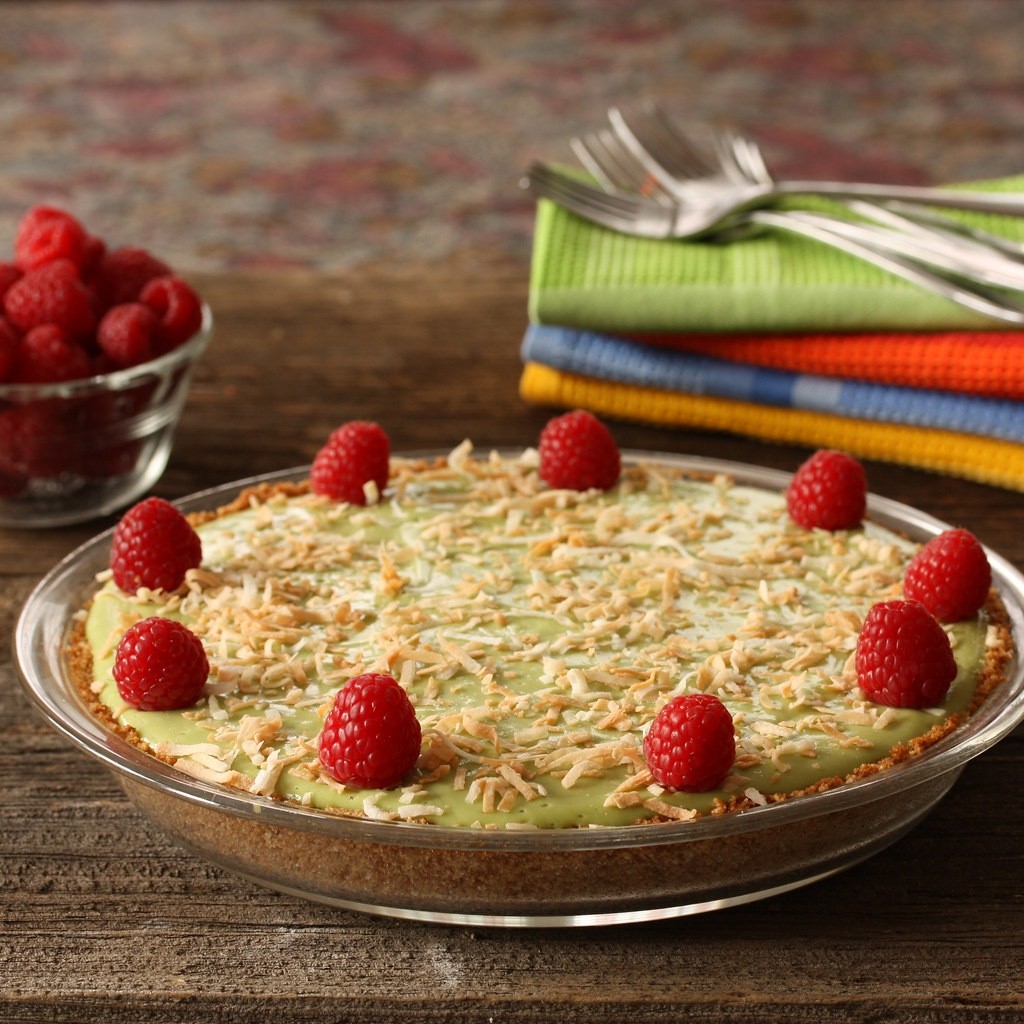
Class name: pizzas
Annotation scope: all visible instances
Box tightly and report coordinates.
[72,412,1017,831]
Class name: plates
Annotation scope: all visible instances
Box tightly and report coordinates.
[8,444,1024,928]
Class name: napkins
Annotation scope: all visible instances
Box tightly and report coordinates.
[514,169,1024,495]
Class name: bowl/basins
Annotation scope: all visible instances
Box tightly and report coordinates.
[1,300,214,526]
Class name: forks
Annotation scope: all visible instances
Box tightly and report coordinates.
[510,99,1022,328]
